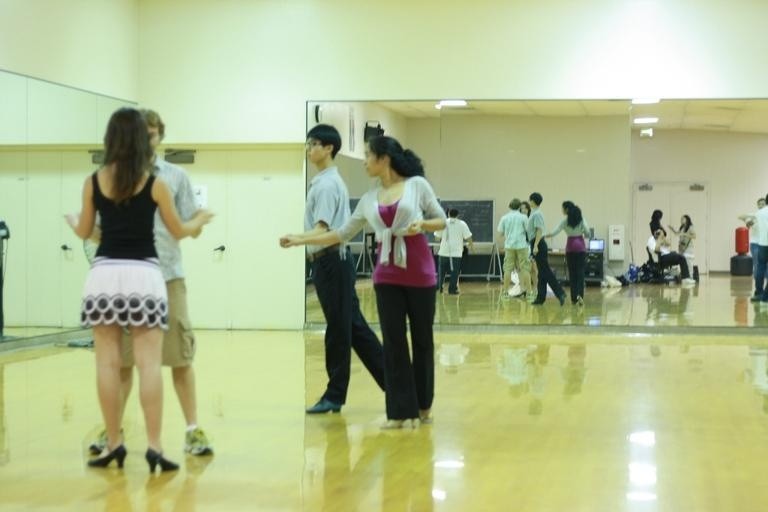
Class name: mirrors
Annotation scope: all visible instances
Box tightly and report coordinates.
[1,68,138,343]
[306,99,768,328]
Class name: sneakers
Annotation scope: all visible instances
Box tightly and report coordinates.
[681,278,695,285]
[184,428,213,455]
[90,429,127,454]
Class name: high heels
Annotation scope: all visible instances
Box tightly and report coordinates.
[381,415,415,430]
[576,295,584,306]
[88,446,126,468]
[419,407,435,422]
[146,449,179,472]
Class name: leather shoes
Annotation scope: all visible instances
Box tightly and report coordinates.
[448,291,459,294]
[558,293,567,305]
[306,398,342,414]
[749,296,762,302]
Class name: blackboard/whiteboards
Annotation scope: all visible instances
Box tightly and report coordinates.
[349,198,364,243]
[423,201,494,244]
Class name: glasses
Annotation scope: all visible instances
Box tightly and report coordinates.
[305,142,322,147]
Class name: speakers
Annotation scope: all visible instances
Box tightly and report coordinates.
[584,252,603,286]
[365,126,384,143]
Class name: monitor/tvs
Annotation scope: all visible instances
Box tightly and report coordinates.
[590,240,604,250]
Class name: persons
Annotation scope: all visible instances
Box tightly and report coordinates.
[497,192,590,306]
[288,134,449,430]
[647,210,697,284]
[278,123,389,413]
[743,195,767,302]
[62,108,213,473]
[435,208,473,296]
[87,109,212,456]
